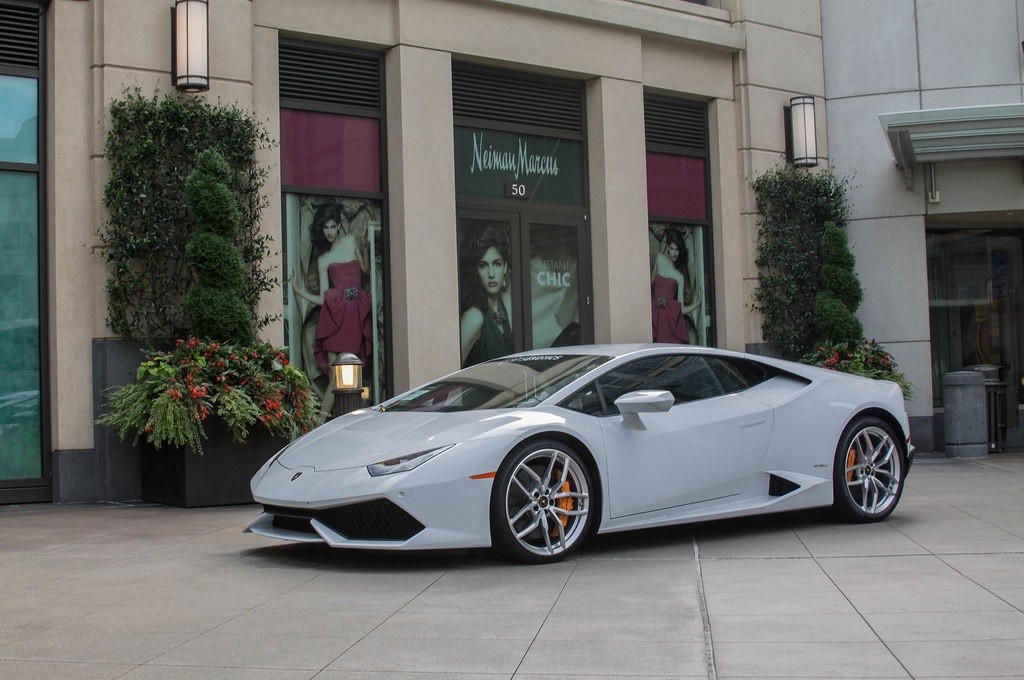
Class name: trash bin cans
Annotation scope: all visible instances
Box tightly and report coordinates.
[962,364,1006,454]
[942,371,988,457]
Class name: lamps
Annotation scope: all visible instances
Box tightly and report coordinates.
[782,96,817,168]
[169,0,210,93]
[330,352,364,390]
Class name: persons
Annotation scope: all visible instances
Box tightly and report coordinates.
[288,204,373,426]
[650,229,702,344]
[459,224,513,370]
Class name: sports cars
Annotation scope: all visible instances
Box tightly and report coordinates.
[1,391,38,434]
[242,342,914,566]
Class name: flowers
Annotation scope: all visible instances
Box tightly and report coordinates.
[94,336,332,456]
[802,338,915,402]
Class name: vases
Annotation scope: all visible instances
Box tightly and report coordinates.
[137,414,289,509]
